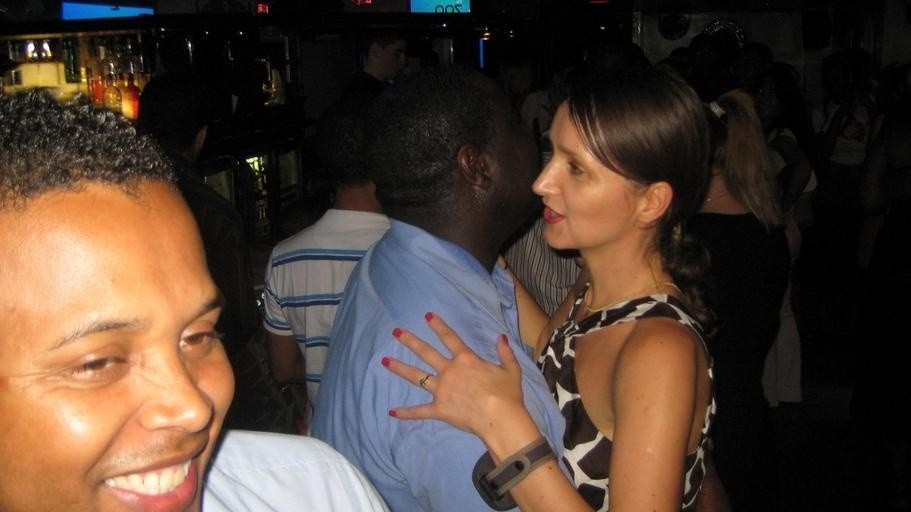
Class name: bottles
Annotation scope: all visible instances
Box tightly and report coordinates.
[88,70,143,122]
[62,37,83,84]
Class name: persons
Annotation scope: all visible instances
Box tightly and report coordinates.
[298,52,732,511]
[122,1,911,450]
[376,57,720,510]
[3,79,395,510]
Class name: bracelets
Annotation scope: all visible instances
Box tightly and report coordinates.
[472,437,558,510]
